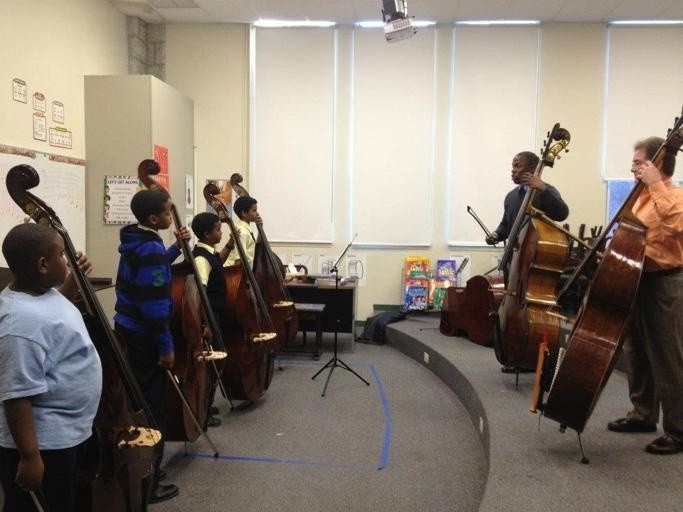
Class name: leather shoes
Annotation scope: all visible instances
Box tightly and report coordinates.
[148,483,178,503]
[209,406,219,414]
[609,416,658,434]
[207,415,221,427]
[158,469,168,479]
[648,432,683,455]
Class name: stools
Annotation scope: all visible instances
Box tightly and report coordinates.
[560,275,587,315]
[294,304,326,361]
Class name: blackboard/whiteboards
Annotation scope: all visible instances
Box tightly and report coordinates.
[602,20,683,184]
[349,21,439,249]
[248,23,337,246]
[445,21,541,246]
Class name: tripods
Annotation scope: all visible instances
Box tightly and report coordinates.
[311,244,370,397]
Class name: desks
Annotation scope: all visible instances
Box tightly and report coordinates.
[283,276,358,347]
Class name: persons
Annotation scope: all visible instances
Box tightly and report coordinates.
[486,152,569,373]
[113,190,191,503]
[224,196,262,271]
[596,137,683,455]
[0,223,102,512]
[192,212,241,426]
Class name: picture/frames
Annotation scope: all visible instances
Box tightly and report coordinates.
[206,178,232,223]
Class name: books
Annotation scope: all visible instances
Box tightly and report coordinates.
[434,260,456,309]
[405,256,429,311]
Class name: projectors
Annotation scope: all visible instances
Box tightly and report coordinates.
[381,0,416,44]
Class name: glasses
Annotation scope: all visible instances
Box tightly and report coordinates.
[631,160,647,168]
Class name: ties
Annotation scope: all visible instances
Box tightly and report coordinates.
[518,187,527,204]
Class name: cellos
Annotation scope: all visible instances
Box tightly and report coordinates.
[491,116,572,374]
[137,158,226,444]
[228,171,302,356]
[5,163,165,510]
[201,181,277,404]
[439,272,507,348]
[539,108,683,433]
